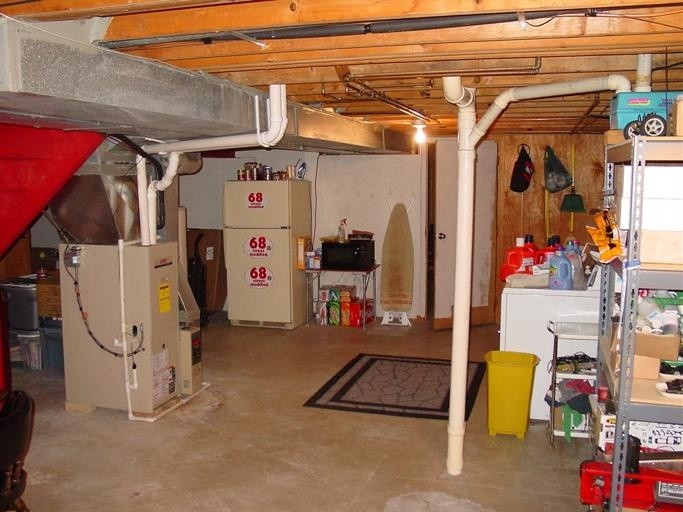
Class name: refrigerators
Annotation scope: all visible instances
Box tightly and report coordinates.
[223,182,314,332]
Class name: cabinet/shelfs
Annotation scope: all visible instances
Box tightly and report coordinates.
[546,319,598,453]
[588,137,682,511]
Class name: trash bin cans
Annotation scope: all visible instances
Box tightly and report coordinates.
[483,350,540,439]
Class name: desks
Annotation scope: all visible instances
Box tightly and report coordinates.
[300,264,382,332]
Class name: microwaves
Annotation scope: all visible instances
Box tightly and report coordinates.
[322,239,372,269]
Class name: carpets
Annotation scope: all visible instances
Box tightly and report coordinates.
[302,352,487,422]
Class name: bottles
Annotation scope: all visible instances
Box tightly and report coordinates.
[237,170,245,181]
[264,167,271,180]
[308,248,322,269]
[337,218,347,244]
[252,168,258,180]
[245,170,252,181]
[636,296,663,329]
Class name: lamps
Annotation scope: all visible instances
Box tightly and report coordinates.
[412,118,427,144]
